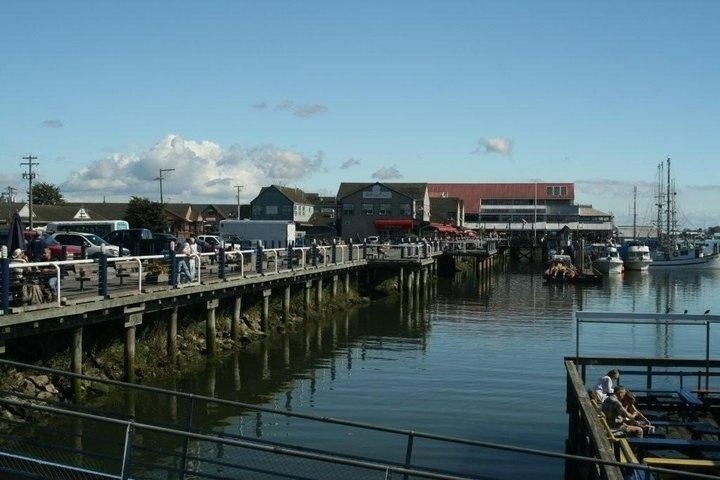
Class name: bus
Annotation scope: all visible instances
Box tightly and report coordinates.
[47,221,130,238]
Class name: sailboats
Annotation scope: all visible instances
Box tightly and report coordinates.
[625,157,720,270]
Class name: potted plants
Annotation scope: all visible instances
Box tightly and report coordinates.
[143,262,171,285]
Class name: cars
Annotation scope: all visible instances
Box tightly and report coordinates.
[366,236,380,246]
[1,229,253,263]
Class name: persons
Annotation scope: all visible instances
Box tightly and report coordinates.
[175,234,201,285]
[603,386,651,438]
[597,369,619,395]
[10,247,31,297]
[38,248,58,302]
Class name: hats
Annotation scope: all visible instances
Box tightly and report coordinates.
[11,248,25,258]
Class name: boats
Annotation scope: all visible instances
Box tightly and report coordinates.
[540,247,625,281]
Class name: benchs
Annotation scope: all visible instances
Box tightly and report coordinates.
[591,391,630,450]
